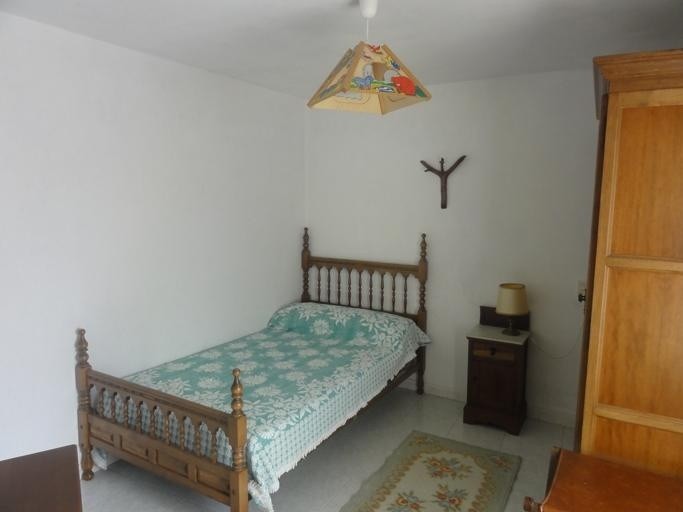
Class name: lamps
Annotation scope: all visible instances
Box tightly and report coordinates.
[307,0,432,115]
[496,283,528,335]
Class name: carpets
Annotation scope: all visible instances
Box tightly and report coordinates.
[339,430,523,512]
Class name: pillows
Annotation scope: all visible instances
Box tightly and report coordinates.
[268,302,415,349]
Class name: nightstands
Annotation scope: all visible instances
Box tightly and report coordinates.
[463,306,532,435]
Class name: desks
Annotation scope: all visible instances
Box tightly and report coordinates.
[523,447,683,512]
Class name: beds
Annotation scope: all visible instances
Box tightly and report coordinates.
[74,227,428,512]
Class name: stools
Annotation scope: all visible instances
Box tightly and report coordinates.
[0,445,82,512]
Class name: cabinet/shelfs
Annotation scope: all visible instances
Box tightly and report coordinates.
[574,49,683,479]
